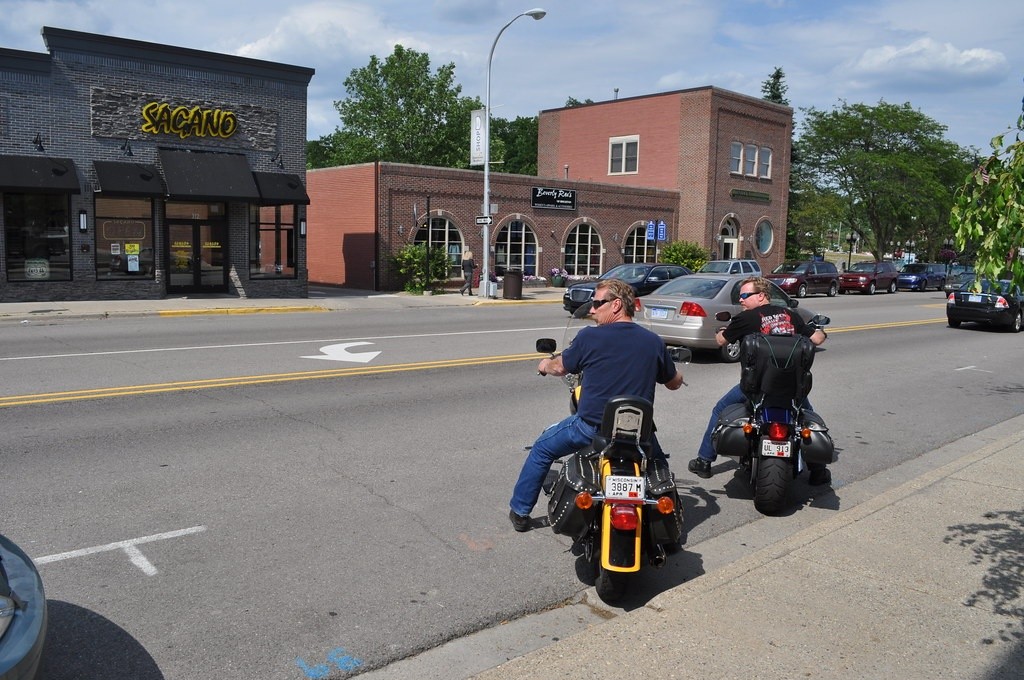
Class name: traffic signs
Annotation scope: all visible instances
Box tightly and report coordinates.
[474,215,494,226]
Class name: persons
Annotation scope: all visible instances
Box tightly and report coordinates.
[687,276,832,486]
[508,279,682,531]
[460,251,478,296]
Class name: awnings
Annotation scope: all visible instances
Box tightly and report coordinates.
[0,155,81,195]
[157,146,260,201]
[252,171,310,205]
[92,161,165,198]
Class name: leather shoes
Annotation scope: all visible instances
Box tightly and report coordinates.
[509,509,529,532]
[689,457,711,478]
[809,469,831,485]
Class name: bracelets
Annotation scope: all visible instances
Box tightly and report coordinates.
[720,328,726,331]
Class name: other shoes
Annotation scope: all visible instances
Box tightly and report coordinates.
[469,293,473,296]
[459,289,464,296]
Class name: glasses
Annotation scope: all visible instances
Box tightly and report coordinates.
[593,297,619,309]
[739,292,759,299]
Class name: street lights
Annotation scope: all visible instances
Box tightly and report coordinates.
[482,8,548,298]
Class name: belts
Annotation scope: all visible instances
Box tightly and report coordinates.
[580,417,601,430]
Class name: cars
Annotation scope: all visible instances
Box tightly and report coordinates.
[896,263,948,291]
[764,260,841,298]
[632,273,824,363]
[561,263,697,319]
[694,259,763,279]
[0,532,49,680]
[946,280,1024,334]
[838,261,899,295]
[944,272,985,299]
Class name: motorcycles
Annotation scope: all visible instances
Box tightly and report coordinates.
[533,301,688,604]
[713,311,834,515]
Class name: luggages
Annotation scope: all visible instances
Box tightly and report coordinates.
[740,332,815,405]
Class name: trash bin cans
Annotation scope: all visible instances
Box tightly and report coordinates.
[503,270,525,300]
